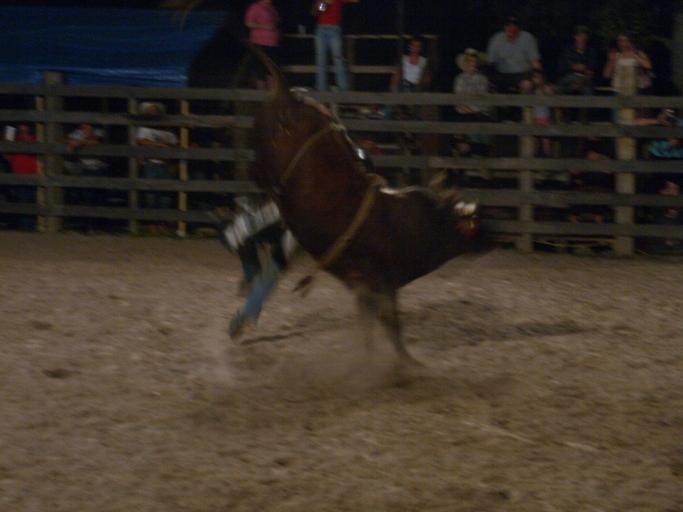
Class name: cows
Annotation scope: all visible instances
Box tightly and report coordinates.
[226,50,496,368]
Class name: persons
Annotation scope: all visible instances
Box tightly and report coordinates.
[216,192,302,341]
[1,1,681,259]
